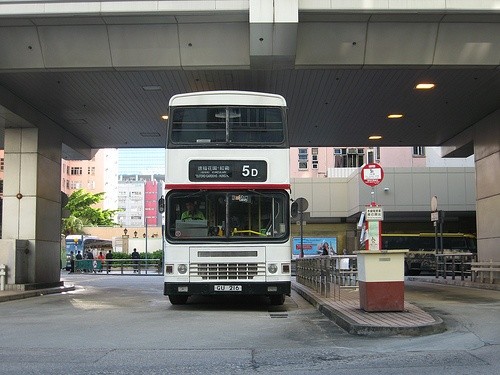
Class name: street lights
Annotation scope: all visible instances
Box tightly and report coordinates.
[121,228,129,255]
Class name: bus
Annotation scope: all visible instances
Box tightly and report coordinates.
[64,234,112,271]
[156,90,299,306]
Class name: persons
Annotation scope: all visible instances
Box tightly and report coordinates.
[132,248,140,273]
[106,250,114,272]
[322,242,329,255]
[181,199,205,221]
[342,249,348,255]
[68,249,105,274]
